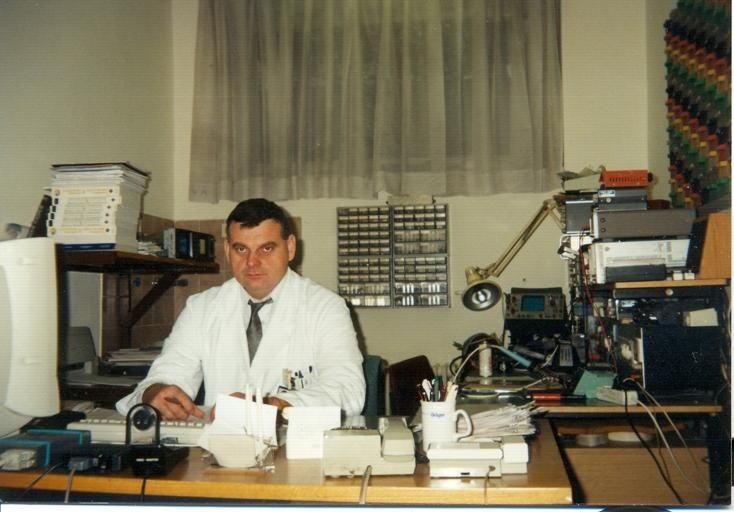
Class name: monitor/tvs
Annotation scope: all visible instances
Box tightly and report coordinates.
[0,234,61,442]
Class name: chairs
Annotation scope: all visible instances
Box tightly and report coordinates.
[379,357,436,421]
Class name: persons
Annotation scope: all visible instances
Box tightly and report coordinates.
[115,199,365,421]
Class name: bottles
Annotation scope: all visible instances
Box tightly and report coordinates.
[477,340,493,378]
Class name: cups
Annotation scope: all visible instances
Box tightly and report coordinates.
[419,399,472,452]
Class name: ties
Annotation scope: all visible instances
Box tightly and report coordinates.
[246,298,273,366]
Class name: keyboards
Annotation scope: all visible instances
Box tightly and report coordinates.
[67,414,207,447]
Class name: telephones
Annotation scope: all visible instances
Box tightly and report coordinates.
[322,416,417,477]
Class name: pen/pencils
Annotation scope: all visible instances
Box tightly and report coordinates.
[414,374,460,401]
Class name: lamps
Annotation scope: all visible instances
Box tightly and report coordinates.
[450,192,558,311]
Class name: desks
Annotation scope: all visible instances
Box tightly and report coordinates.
[447,358,730,421]
[3,401,575,508]
